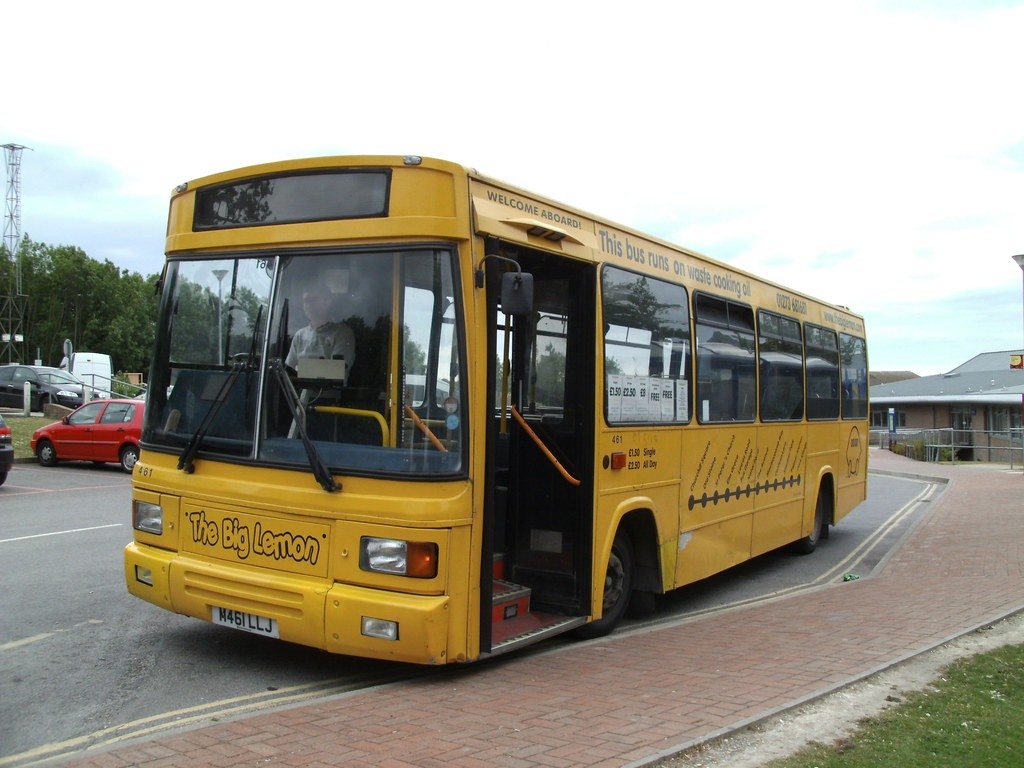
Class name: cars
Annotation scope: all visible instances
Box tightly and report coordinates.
[0,363,106,412]
[0,415,15,487]
[30,399,146,474]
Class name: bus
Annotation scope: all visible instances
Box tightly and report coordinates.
[120,147,874,673]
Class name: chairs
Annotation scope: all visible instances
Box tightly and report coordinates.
[110,411,125,422]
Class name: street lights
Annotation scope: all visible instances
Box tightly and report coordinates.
[1012,254,1024,323]
[211,268,229,365]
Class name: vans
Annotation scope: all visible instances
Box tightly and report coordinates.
[404,374,451,408]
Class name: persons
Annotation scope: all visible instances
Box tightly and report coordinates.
[278,283,358,440]
